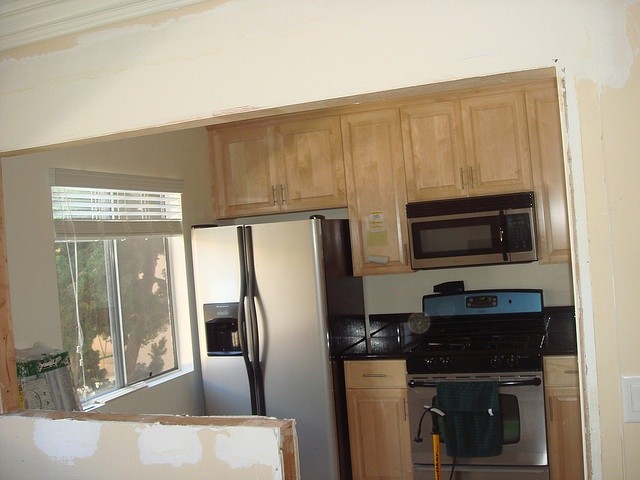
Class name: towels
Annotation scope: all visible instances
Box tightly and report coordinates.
[436,381,505,458]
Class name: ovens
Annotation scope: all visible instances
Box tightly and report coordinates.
[405,370,549,480]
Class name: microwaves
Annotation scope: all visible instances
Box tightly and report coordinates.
[405,191,538,270]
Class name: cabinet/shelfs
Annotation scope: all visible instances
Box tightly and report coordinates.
[339,108,412,277]
[542,355,586,480]
[524,88,572,266]
[397,89,534,199]
[206,107,348,220]
[342,359,413,479]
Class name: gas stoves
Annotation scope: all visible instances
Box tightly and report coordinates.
[406,328,548,373]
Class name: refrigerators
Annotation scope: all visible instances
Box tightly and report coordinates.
[191,215,367,480]
